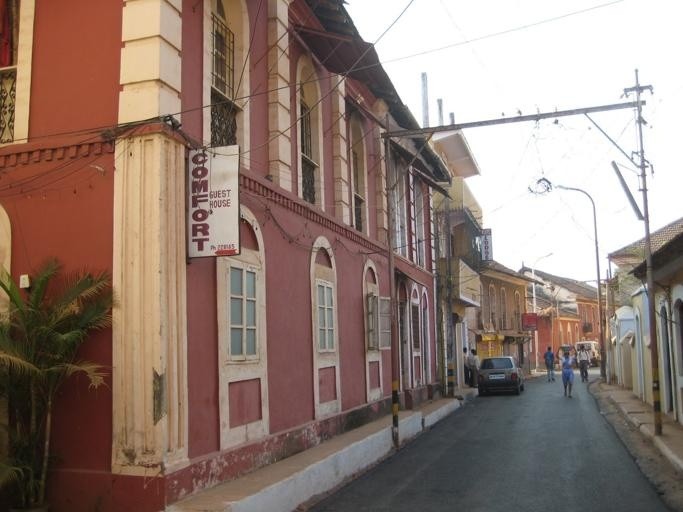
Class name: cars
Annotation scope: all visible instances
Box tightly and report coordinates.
[478,356,524,395]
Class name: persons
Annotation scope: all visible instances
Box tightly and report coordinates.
[463,348,480,388]
[556,345,579,398]
[577,346,590,382]
[544,347,555,383]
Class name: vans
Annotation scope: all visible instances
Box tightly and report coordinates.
[574,341,601,366]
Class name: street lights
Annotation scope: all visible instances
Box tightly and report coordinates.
[537,178,606,377]
